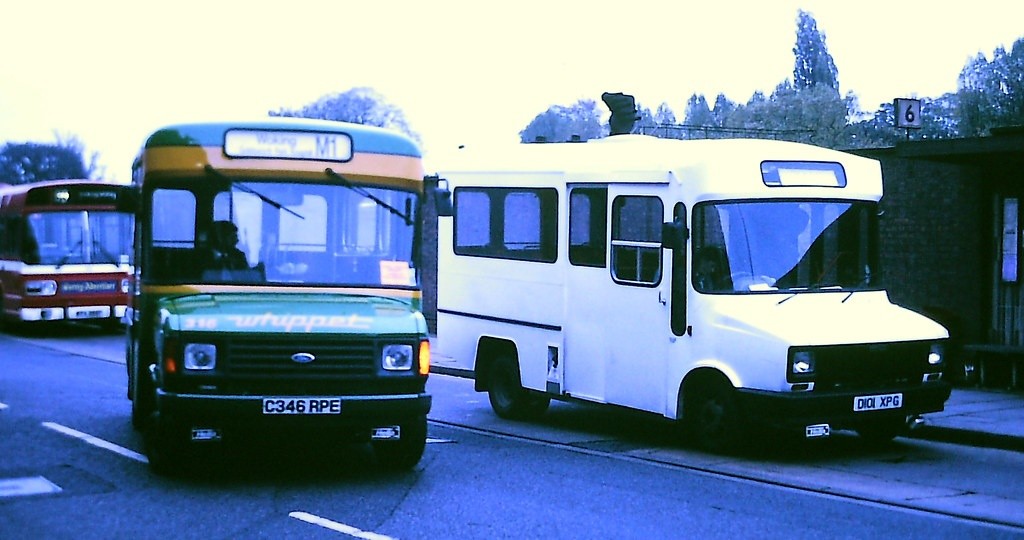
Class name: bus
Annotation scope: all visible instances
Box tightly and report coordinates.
[0,175,131,337]
[124,117,434,476]
[438,130,949,457]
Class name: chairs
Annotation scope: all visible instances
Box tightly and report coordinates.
[484,240,805,295]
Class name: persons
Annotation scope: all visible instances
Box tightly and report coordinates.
[183,220,250,285]
